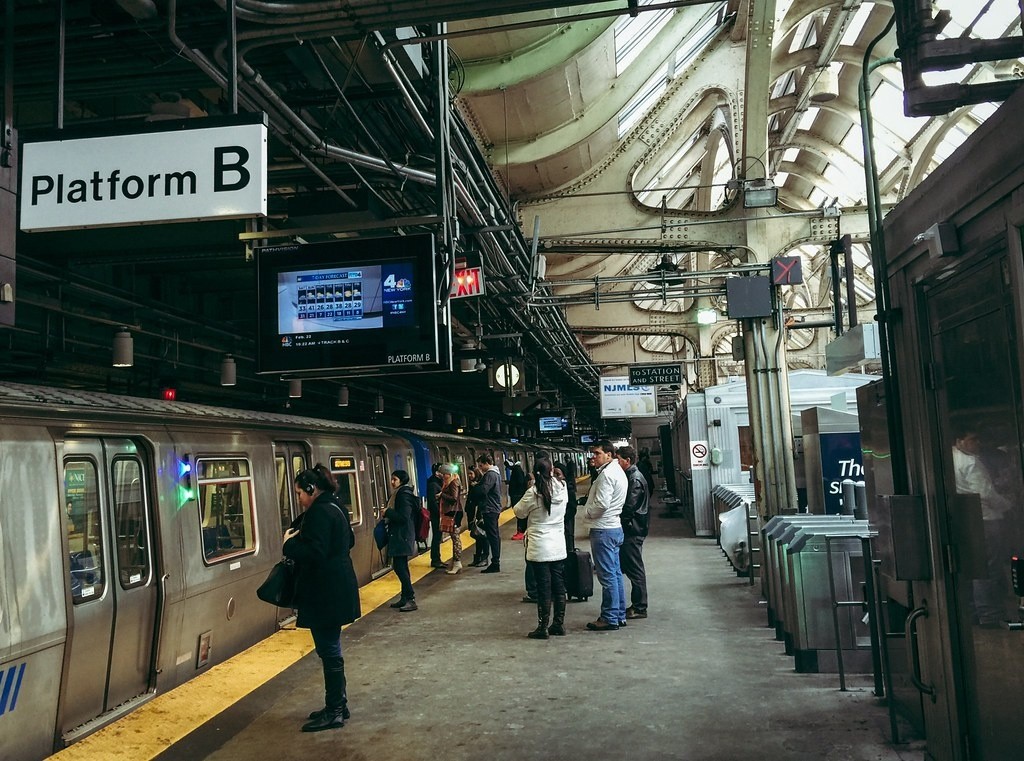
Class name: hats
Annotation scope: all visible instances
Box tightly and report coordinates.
[439,464,453,474]
[432,463,443,474]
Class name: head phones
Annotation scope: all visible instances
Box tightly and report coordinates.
[302,470,315,495]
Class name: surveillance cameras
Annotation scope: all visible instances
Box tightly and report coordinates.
[474,363,486,373]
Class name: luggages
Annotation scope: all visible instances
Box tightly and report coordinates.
[566,548,593,601]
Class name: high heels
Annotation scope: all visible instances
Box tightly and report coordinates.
[447,561,462,574]
[445,561,455,573]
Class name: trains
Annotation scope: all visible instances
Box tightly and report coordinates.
[0,381,594,761]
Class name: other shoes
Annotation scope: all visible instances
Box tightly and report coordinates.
[619,620,626,626]
[390,597,408,608]
[626,607,647,619]
[476,559,488,566]
[481,564,500,572]
[431,560,449,568]
[523,596,535,603]
[468,561,481,566]
[587,617,619,630]
[399,598,417,612]
[511,533,524,540]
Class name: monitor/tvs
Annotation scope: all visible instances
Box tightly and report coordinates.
[534,409,599,446]
[254,233,453,381]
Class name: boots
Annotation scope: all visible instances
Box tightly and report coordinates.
[302,658,344,732]
[549,601,566,635]
[528,604,551,639]
[310,658,350,719]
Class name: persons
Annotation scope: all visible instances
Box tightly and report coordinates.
[427,463,464,574]
[380,470,422,611]
[284,465,361,731]
[504,451,577,638]
[584,441,655,629]
[952,427,1024,623]
[465,456,503,572]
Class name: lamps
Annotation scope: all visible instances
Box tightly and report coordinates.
[403,403,411,418]
[513,427,517,436]
[112,326,133,367]
[337,383,348,406]
[485,420,490,431]
[527,429,531,437]
[220,354,236,386]
[495,423,500,432]
[520,429,524,435]
[445,413,452,424]
[474,419,479,429]
[743,179,778,209]
[289,380,302,397]
[505,425,509,434]
[460,343,477,373]
[375,396,384,413]
[426,408,433,422]
[460,416,466,427]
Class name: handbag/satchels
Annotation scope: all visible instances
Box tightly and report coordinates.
[256,558,298,607]
[440,516,455,532]
[414,498,430,542]
[470,506,487,540]
[374,520,388,552]
